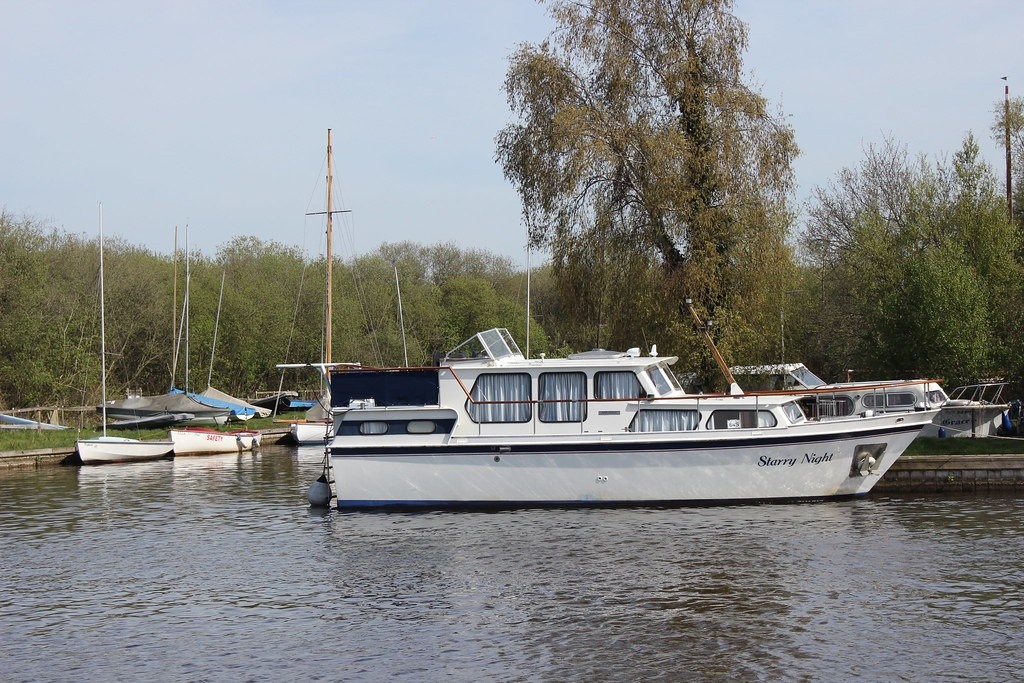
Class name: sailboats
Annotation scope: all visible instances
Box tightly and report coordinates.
[74,202,175,461]
[271,129,384,446]
[96,225,299,429]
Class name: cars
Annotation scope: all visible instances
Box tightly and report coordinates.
[727,363,826,393]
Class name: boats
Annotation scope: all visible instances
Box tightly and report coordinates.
[169,427,262,456]
[326,201,936,508]
[782,379,1011,437]
